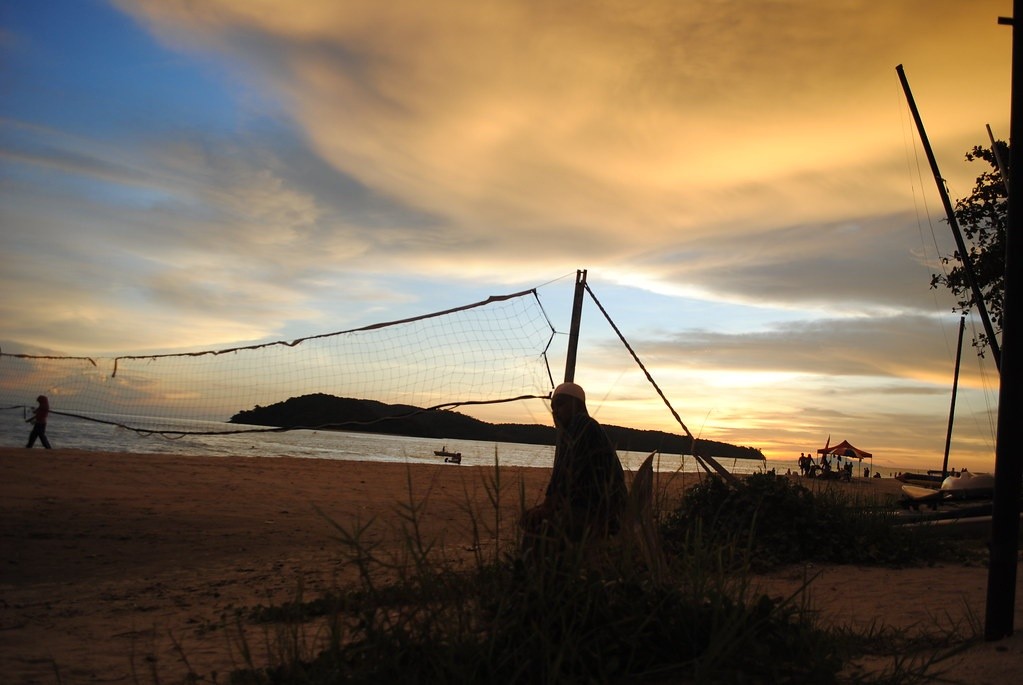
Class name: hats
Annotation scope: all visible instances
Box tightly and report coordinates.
[551,384,585,402]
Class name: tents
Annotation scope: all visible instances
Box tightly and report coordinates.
[817,441,872,479]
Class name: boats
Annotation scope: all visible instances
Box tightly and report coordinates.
[434,446,457,457]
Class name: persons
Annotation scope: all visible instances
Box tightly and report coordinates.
[863,467,869,477]
[821,456,831,479]
[24,396,52,447]
[772,467,775,476]
[798,453,815,478]
[785,468,791,475]
[521,384,627,566]
[873,472,880,478]
[949,467,968,477]
[844,461,853,477]
